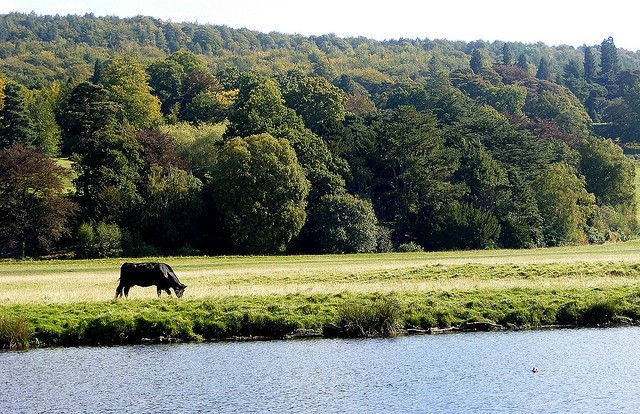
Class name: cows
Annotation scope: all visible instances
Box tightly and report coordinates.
[114,262,187,300]
